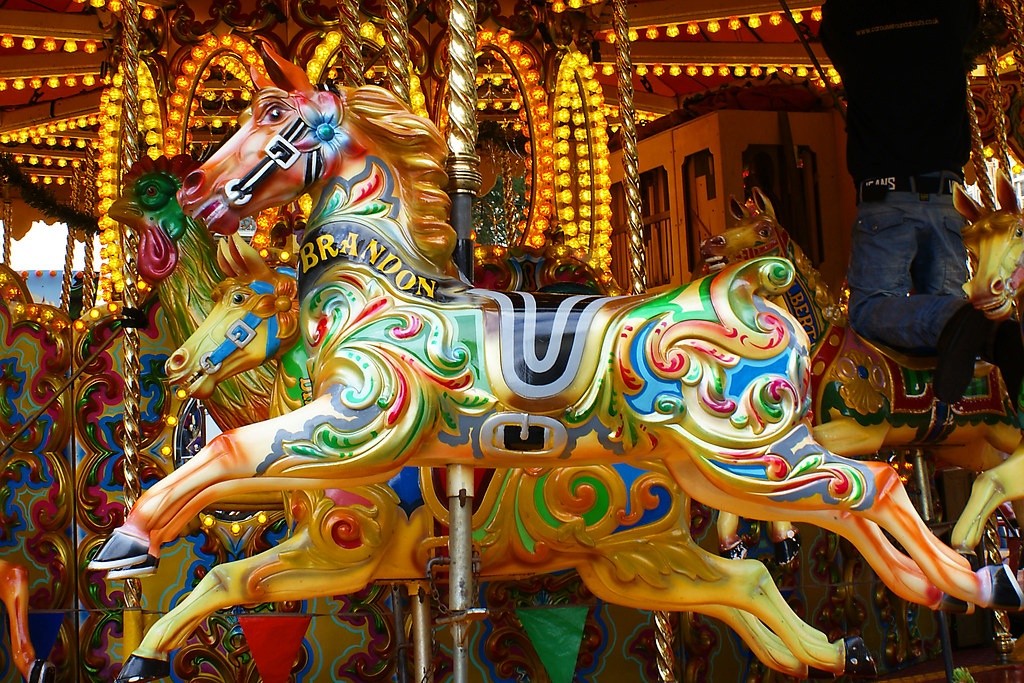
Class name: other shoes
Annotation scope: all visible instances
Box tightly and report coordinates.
[992,317,1024,413]
[933,309,983,402]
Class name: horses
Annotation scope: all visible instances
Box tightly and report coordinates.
[87,41,1024,683]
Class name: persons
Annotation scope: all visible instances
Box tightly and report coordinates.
[818,0,1024,412]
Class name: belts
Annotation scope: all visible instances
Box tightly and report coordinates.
[859,176,963,202]
